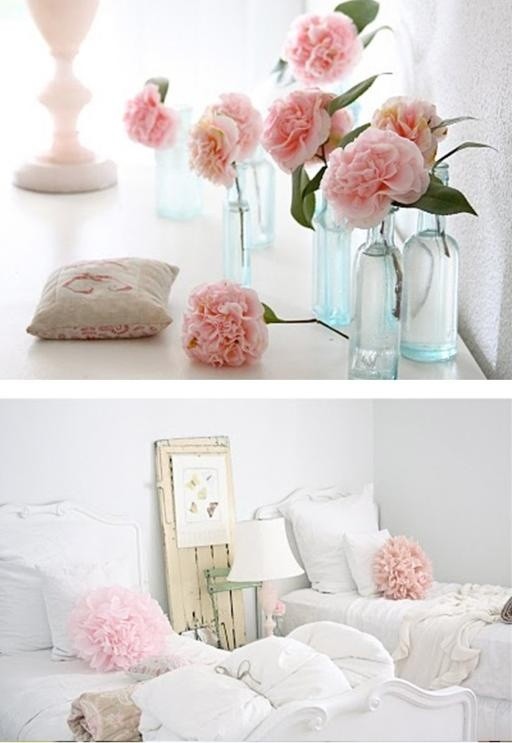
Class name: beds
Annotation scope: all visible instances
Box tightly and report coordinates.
[253,487,512,742]
[0,501,477,742]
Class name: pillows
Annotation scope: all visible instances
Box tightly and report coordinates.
[26,256,180,340]
[0,550,190,682]
[278,494,433,599]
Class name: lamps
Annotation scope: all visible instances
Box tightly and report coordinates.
[226,517,305,637]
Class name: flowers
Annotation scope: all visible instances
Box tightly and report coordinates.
[122,1,498,380]
[272,599,286,636]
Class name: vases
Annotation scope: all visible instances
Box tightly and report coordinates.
[272,616,285,637]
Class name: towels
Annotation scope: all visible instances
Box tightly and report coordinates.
[67,621,394,741]
